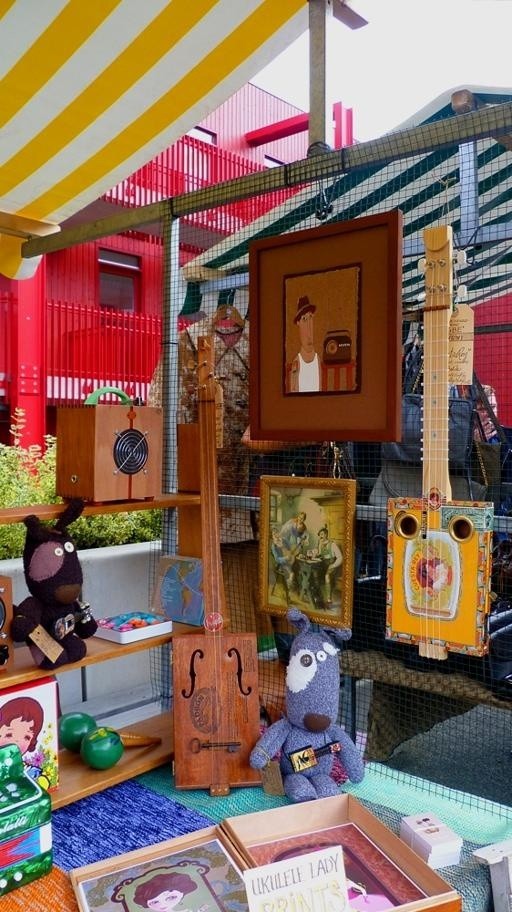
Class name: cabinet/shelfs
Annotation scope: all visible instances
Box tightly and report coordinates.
[1,422,234,813]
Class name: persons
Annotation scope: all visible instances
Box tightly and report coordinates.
[2,696,48,790]
[133,871,209,911]
[289,295,323,395]
[280,511,310,557]
[315,528,343,604]
[270,522,311,604]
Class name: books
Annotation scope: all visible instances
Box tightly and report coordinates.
[149,554,219,628]
[399,811,463,870]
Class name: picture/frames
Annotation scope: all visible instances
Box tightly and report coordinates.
[69,824,252,911]
[256,473,357,631]
[247,208,403,443]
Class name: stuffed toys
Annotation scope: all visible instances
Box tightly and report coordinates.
[9,495,98,670]
[249,603,364,803]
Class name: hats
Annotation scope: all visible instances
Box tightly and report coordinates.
[317,527,329,537]
[293,295,317,324]
